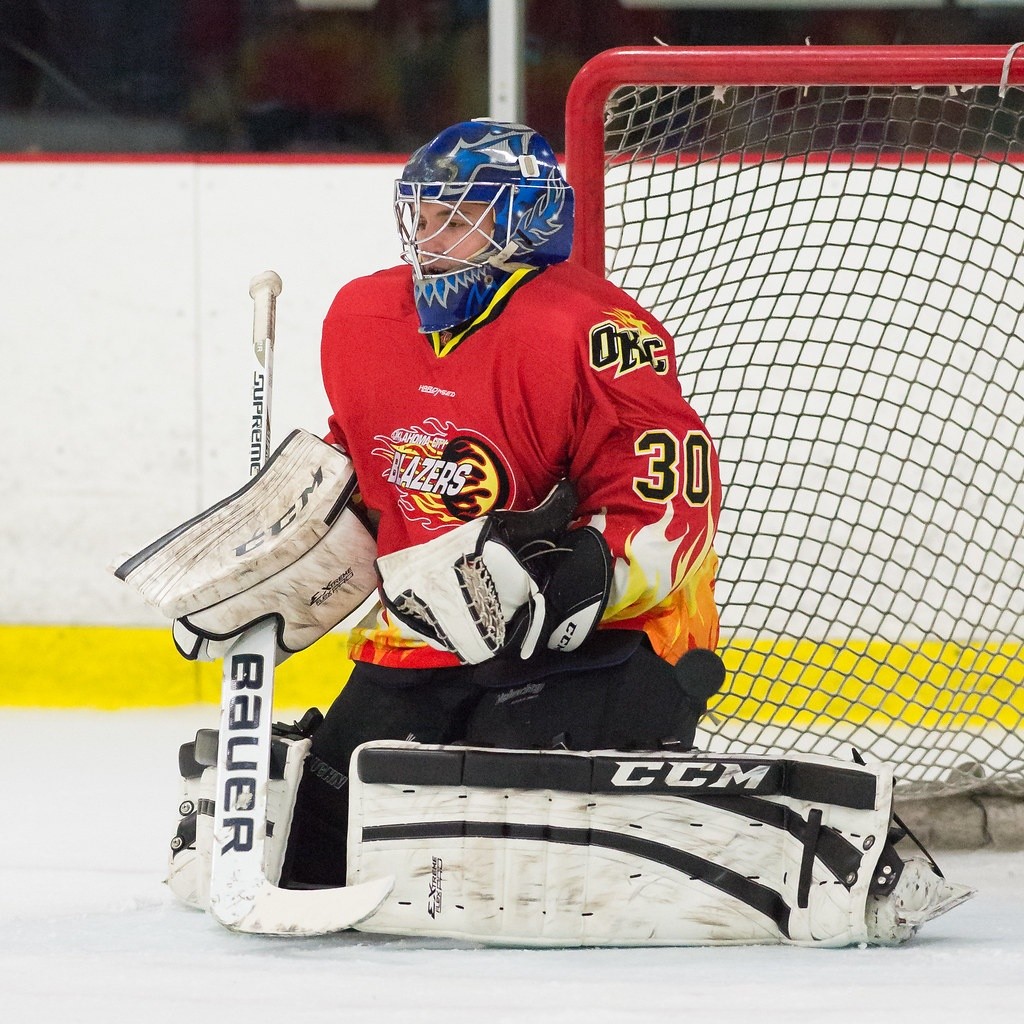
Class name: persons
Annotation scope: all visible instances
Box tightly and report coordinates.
[167,117,946,946]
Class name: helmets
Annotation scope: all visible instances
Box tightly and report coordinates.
[393,116,575,333]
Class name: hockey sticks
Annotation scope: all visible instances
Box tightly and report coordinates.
[206,271,398,941]
